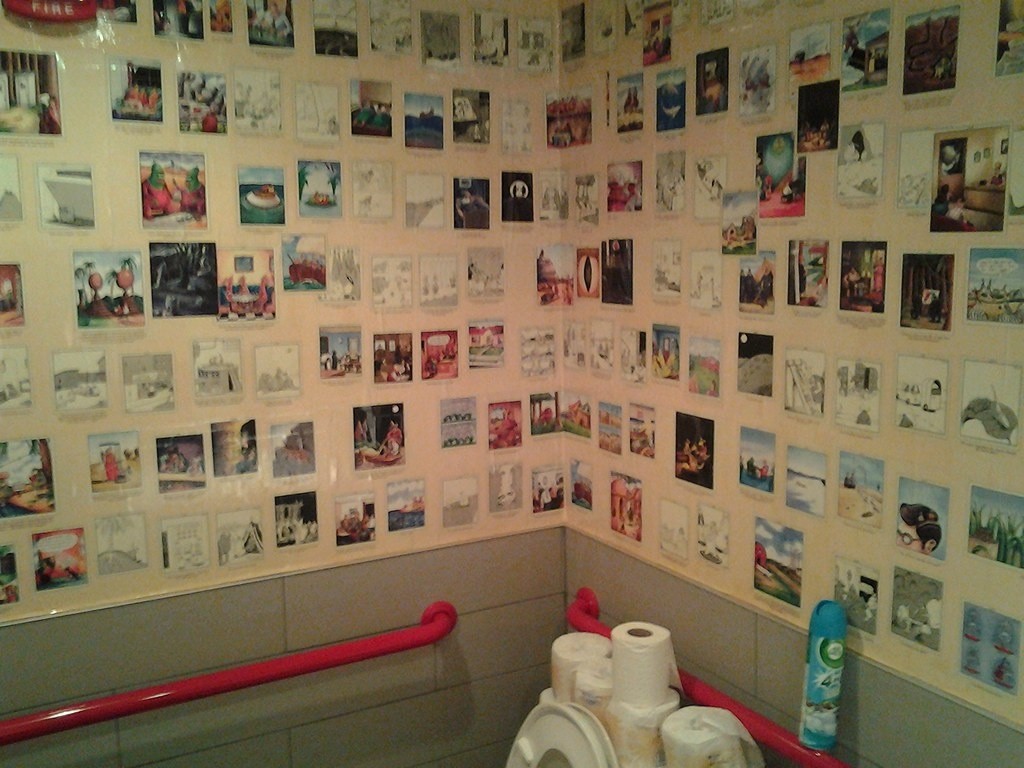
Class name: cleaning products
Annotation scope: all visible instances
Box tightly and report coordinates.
[796,599,847,753]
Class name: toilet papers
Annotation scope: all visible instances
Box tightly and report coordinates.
[550,619,765,768]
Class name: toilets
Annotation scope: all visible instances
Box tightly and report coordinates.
[504,686,622,768]
